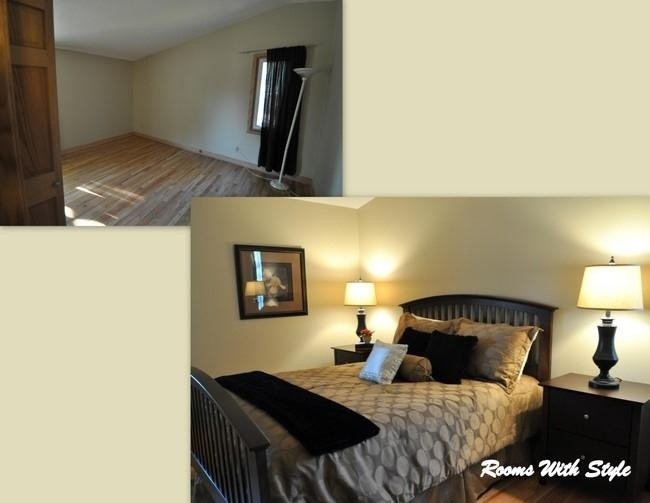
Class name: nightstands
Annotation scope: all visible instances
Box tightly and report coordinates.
[538,372,650,503]
[331,344,369,365]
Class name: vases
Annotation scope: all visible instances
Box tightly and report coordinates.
[363,337,372,344]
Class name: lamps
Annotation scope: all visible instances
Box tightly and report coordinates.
[344,279,376,342]
[269,68,319,191]
[576,257,644,389]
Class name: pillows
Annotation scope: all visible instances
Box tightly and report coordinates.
[425,329,479,384]
[392,311,452,344]
[357,339,409,385]
[399,352,435,381]
[452,317,544,395]
[397,327,430,357]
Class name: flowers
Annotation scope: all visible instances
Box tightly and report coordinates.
[360,328,374,338]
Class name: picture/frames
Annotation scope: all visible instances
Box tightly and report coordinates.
[234,243,308,320]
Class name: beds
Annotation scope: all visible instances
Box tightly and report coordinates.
[191,293,558,503]
